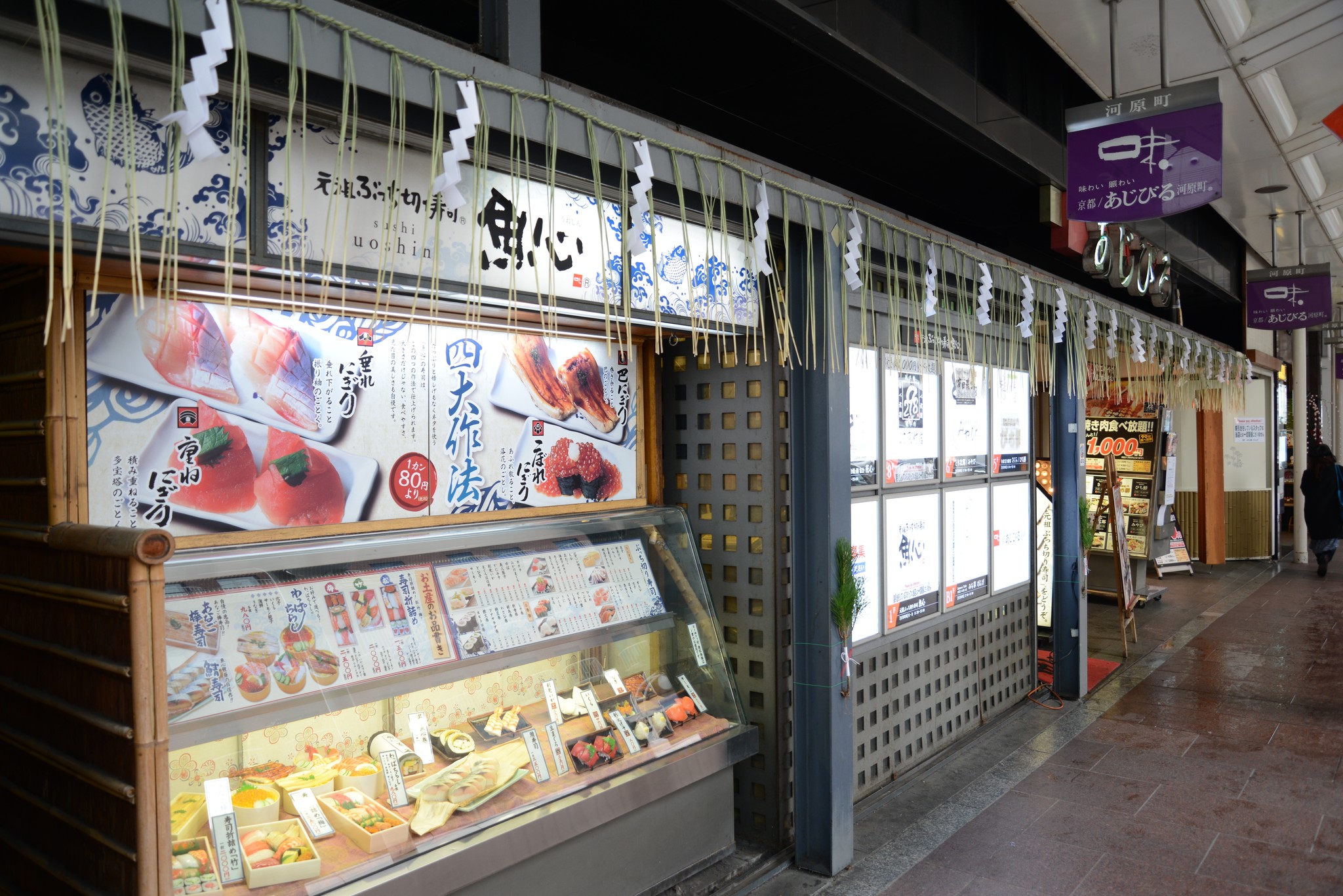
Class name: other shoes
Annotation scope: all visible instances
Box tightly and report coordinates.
[1317,568,1327,577]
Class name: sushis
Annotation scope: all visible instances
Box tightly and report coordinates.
[235,584,410,695]
[443,568,486,655]
[528,557,560,637]
[170,671,707,894]
[165,661,218,714]
[126,299,349,531]
[583,548,615,625]
[517,335,624,499]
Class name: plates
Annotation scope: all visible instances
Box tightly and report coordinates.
[496,417,636,509]
[235,818,321,889]
[490,336,628,447]
[427,738,476,761]
[444,569,470,590]
[555,681,601,722]
[379,583,412,637]
[170,791,214,841]
[623,705,675,748]
[536,618,558,636]
[597,691,642,730]
[406,753,530,812]
[588,566,607,586]
[85,295,369,444]
[361,750,426,784]
[349,588,385,632]
[172,836,222,896]
[526,557,549,576]
[449,589,475,609]
[167,651,231,726]
[532,574,554,595]
[467,704,533,743]
[315,786,410,854]
[164,607,220,656]
[582,551,604,568]
[324,590,359,648]
[116,398,380,529]
[565,725,625,774]
[620,670,657,705]
[658,688,704,728]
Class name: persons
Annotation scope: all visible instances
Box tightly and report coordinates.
[1299,443,1343,576]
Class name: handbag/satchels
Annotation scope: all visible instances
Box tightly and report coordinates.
[1335,464,1343,505]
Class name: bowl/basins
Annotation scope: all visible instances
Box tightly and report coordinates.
[332,757,384,803]
[292,750,316,775]
[308,649,339,685]
[280,625,315,662]
[243,631,277,667]
[236,663,271,702]
[273,656,307,694]
[283,770,333,816]
[229,785,280,828]
[241,776,283,808]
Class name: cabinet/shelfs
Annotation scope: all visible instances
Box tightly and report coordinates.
[1083,405,1176,608]
[160,501,768,896]
